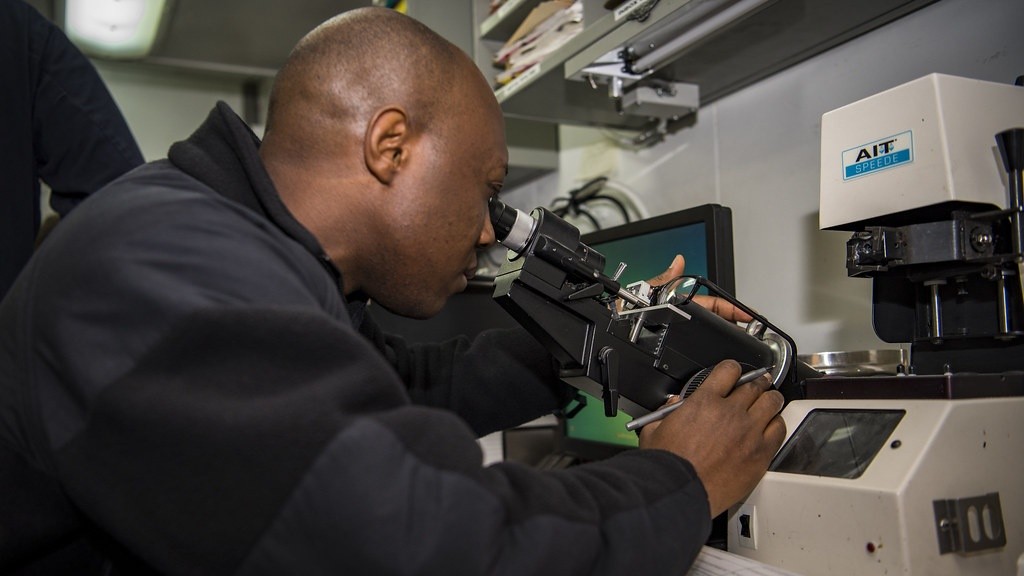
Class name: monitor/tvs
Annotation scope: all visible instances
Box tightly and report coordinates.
[558,203,736,461]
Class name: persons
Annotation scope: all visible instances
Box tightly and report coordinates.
[0,4,787,575]
[0,0,151,289]
